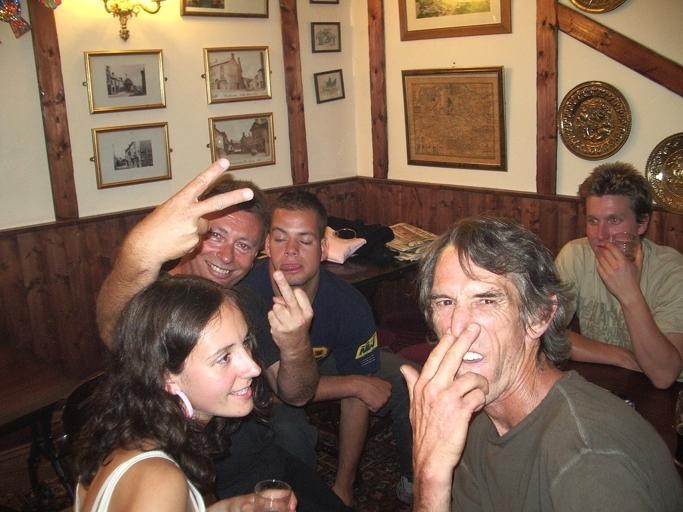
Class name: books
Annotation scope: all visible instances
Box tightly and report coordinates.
[382,220,441,264]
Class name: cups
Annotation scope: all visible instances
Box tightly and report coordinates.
[253,480,292,512]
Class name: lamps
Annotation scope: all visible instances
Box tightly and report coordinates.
[101,0,162,42]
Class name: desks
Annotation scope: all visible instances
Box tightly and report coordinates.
[254,253,427,332]
[1,334,86,512]
[554,359,682,482]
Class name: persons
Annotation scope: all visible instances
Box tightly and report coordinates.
[547,160,682,392]
[94,156,354,512]
[398,212,682,512]
[227,186,393,506]
[60,274,300,511]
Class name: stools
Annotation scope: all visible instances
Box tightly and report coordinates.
[291,399,385,487]
[395,339,439,372]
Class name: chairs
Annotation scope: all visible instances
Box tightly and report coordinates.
[24,370,122,512]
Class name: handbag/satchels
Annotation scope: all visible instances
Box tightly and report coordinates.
[323,227,366,264]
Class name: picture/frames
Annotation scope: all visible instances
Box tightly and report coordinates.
[83,48,168,115]
[180,1,270,19]
[308,0,346,106]
[400,65,509,174]
[396,0,513,42]
[90,121,173,191]
[205,111,277,172]
[202,43,273,106]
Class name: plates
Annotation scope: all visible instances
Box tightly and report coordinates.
[556,79,631,161]
[568,0,626,13]
[643,132,682,215]
